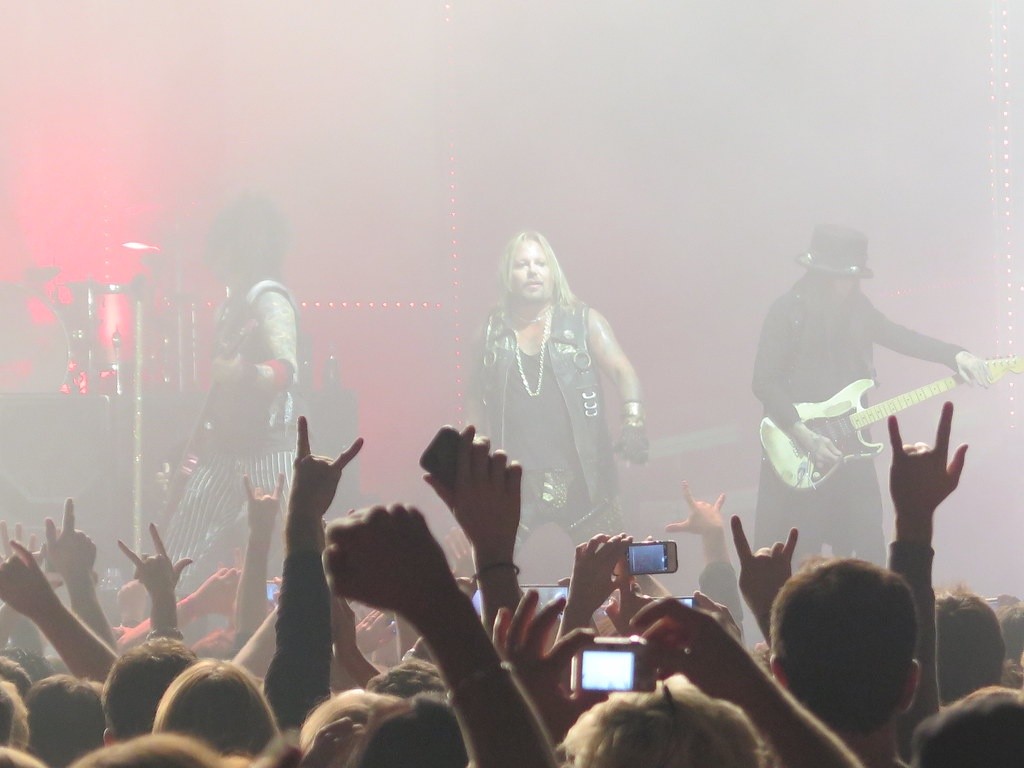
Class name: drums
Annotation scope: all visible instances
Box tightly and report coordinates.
[0,281,72,393]
[54,282,139,396]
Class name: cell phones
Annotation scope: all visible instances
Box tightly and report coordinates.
[267,581,279,601]
[520,584,570,619]
[652,596,694,608]
[613,540,678,576]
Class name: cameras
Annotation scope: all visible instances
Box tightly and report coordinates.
[569,636,657,693]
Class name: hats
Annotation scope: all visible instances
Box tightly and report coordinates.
[799,223,876,284]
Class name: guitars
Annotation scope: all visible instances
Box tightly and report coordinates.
[758,356,1023,490]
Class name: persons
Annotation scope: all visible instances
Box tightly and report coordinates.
[0,401,1024,768]
[154,196,311,599]
[753,226,994,570]
[474,230,645,584]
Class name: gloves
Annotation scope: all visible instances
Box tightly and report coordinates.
[614,417,650,466]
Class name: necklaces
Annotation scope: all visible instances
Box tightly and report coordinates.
[509,310,552,397]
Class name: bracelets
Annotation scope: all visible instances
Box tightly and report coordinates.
[475,563,520,577]
[146,626,184,640]
